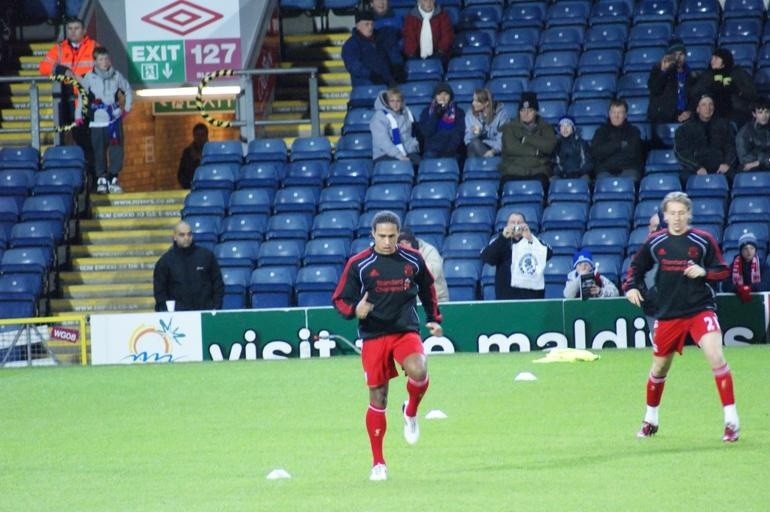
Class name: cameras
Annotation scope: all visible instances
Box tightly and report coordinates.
[671,55,679,64]
[512,224,522,233]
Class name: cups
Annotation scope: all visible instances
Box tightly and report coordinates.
[165,300,176,312]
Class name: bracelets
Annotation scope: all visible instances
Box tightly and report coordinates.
[54,65,69,75]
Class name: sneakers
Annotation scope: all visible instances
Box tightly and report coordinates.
[402,401,419,445]
[107,177,123,194]
[96,177,108,194]
[636,420,659,439]
[723,423,741,442]
[369,464,388,482]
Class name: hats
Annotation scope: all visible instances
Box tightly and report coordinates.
[432,83,453,95]
[558,114,576,131]
[737,228,759,249]
[572,250,593,269]
[518,92,540,112]
[665,37,687,55]
[354,10,376,22]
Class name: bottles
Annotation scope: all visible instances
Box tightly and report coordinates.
[686,259,696,268]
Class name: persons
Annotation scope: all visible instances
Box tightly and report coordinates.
[563,247,621,300]
[37,17,101,161]
[73,45,133,195]
[497,92,556,197]
[152,220,225,314]
[735,97,770,172]
[397,229,450,304]
[367,87,421,162]
[462,87,512,159]
[413,81,466,162]
[646,37,693,151]
[590,99,642,187]
[479,211,554,301]
[330,210,444,482]
[351,0,405,68]
[402,1,455,64]
[340,11,410,90]
[676,47,761,124]
[176,122,210,189]
[643,212,660,294]
[632,190,745,441]
[548,114,594,187]
[674,93,738,186]
[720,231,770,305]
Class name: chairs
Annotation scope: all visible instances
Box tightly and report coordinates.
[0,139,91,319]
